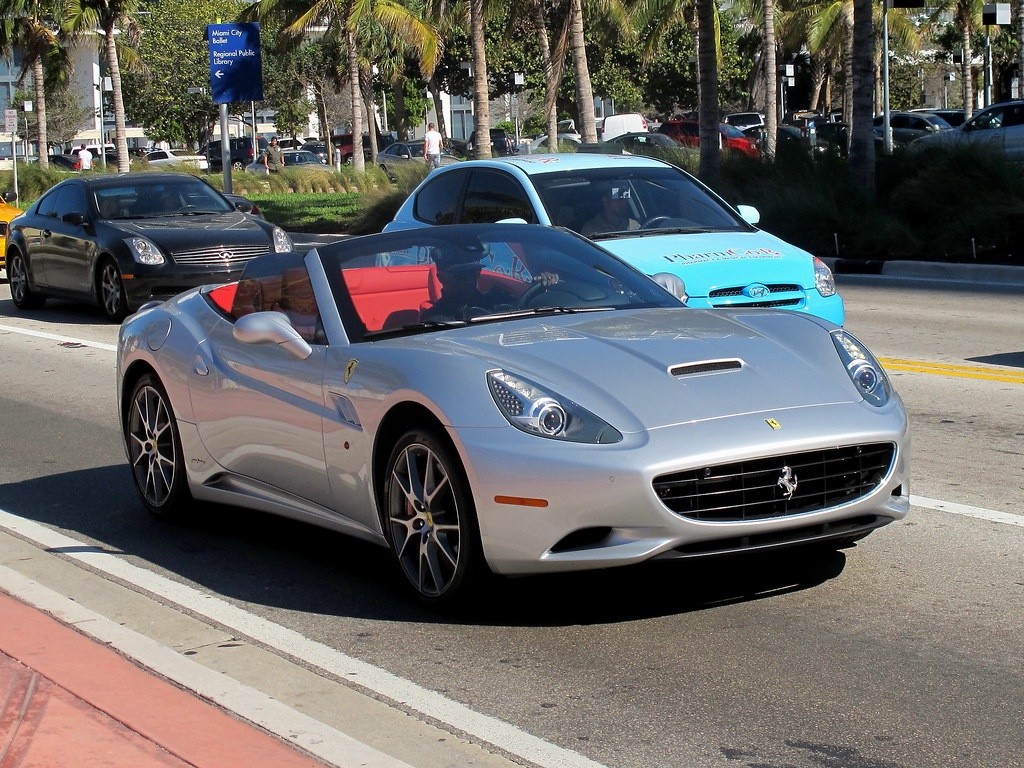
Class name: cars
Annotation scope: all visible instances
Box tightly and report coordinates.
[789,106,982,159]
[1,192,26,270]
[741,126,842,164]
[519,133,582,155]
[269,138,305,154]
[278,151,324,167]
[4,173,298,326]
[141,149,208,176]
[35,154,97,172]
[99,146,151,166]
[603,132,700,170]
[378,140,463,169]
[377,154,844,333]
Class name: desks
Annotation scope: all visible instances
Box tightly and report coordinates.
[246,266,445,331]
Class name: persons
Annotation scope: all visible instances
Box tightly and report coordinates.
[581,181,641,236]
[151,143,156,150]
[77,143,93,175]
[424,123,444,168]
[419,240,560,322]
[264,136,285,173]
[257,135,267,149]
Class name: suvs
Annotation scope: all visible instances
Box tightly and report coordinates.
[658,119,758,163]
[908,99,1024,165]
[330,133,396,167]
[467,129,512,158]
[196,136,269,171]
[722,112,763,136]
[300,139,338,164]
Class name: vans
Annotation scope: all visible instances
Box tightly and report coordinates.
[65,144,116,158]
[558,114,662,144]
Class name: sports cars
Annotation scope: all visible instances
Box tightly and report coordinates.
[109,222,914,616]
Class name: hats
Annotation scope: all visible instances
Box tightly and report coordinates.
[430,243,486,275]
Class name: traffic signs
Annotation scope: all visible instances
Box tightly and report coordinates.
[208,21,266,103]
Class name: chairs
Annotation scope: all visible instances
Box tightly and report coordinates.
[282,267,326,343]
[419,268,478,329]
